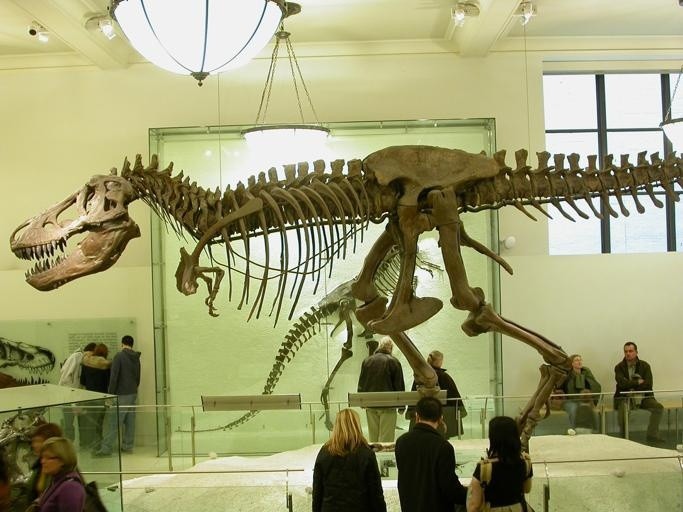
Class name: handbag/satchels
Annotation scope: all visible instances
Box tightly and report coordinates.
[67,476,107,512]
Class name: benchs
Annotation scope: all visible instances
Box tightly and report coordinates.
[519,401,683,439]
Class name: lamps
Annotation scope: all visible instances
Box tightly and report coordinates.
[27,20,48,42]
[83,10,116,40]
[240,1,331,167]
[514,0,537,26]
[450,0,480,28]
[109,0,289,86]
[659,64,683,153]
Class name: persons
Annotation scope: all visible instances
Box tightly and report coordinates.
[28,423,61,502]
[560,354,601,434]
[405,350,467,437]
[35,436,86,512]
[394,396,468,511]
[466,415,533,512]
[357,340,405,442]
[58,335,141,458]
[613,342,666,444]
[312,409,387,512]
